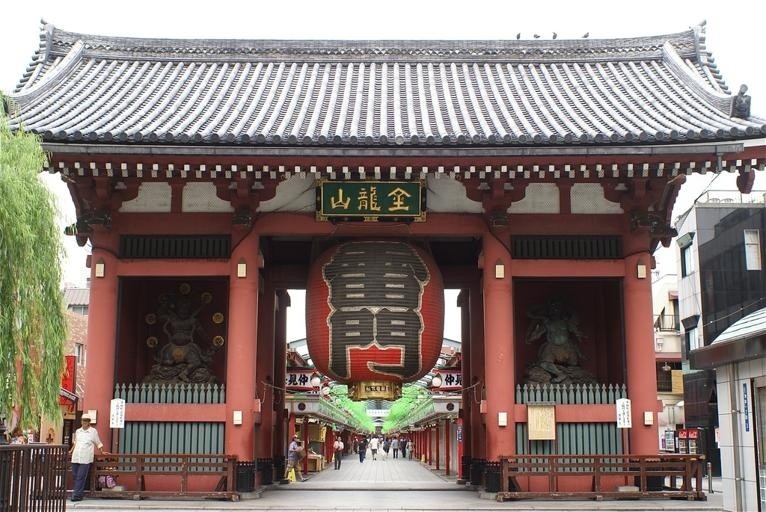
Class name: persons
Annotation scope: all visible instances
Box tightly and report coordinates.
[284,434,309,481]
[333,434,414,470]
[69,414,112,500]
[526,290,592,383]
[145,292,214,381]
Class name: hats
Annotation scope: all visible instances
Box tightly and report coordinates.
[82,413,91,419]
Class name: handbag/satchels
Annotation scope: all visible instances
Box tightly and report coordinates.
[98,476,116,488]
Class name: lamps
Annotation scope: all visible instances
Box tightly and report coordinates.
[256,372,321,404]
[431,373,484,405]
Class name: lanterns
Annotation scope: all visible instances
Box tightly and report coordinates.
[305,239,445,400]
